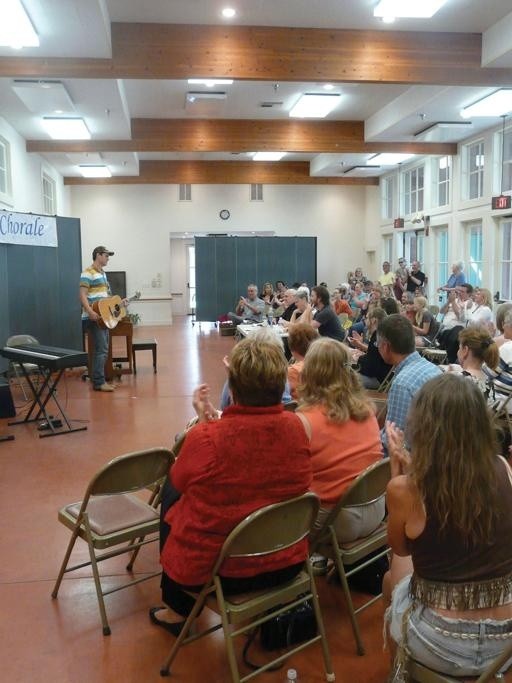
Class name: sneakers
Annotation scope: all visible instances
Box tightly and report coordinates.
[94,383,115,391]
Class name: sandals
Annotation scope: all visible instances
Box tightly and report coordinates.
[309,557,329,576]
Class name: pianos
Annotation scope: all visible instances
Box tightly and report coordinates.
[1,341,90,369]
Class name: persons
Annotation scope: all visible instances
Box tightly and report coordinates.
[380,371,512,681]
[292,336,386,575]
[80,245,130,391]
[149,340,311,636]
[222,256,512,461]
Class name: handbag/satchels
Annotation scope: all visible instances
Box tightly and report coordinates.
[260,598,317,651]
[337,547,389,596]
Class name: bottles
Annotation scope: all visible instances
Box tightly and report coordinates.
[285,668,298,682]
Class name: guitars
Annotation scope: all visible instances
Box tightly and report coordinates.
[91,289,142,330]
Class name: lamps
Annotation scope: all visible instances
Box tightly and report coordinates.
[41,118,91,141]
[462,88,512,119]
[221,9,236,19]
[78,165,112,178]
[287,93,341,119]
[343,167,381,178]
[188,78,233,86]
[251,151,289,163]
[12,80,78,115]
[372,0,448,20]
[0,0,40,50]
[367,154,413,165]
[414,123,474,145]
[182,91,228,115]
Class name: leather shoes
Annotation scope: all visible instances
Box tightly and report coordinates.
[150,607,189,639]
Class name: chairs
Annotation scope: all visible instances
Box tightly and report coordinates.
[6,334,52,400]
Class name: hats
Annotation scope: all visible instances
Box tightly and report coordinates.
[93,246,114,255]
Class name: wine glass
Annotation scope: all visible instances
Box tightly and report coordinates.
[262,310,274,325]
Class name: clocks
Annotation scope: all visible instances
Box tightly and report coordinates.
[220,210,230,220]
